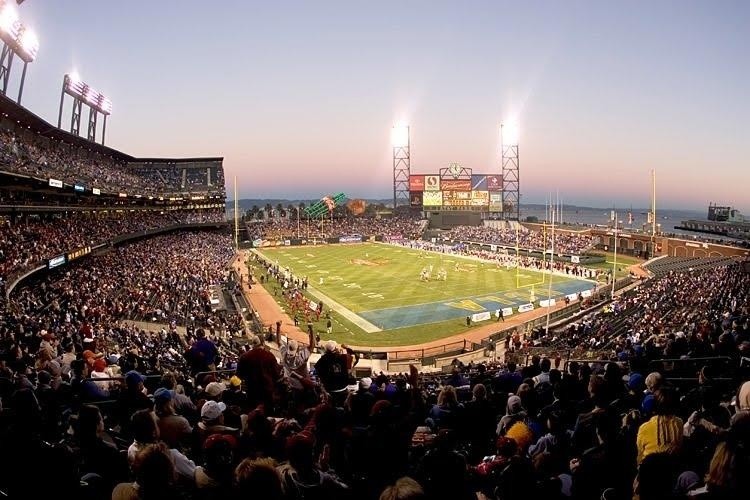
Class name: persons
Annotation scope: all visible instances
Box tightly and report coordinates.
[0,116,750,500]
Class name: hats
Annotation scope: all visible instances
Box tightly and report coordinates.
[83,350,121,372]
[577,365,591,375]
[701,366,717,380]
[287,340,298,355]
[361,377,372,389]
[324,340,336,352]
[125,370,243,448]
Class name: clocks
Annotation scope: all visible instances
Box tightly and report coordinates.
[449,163,461,175]
